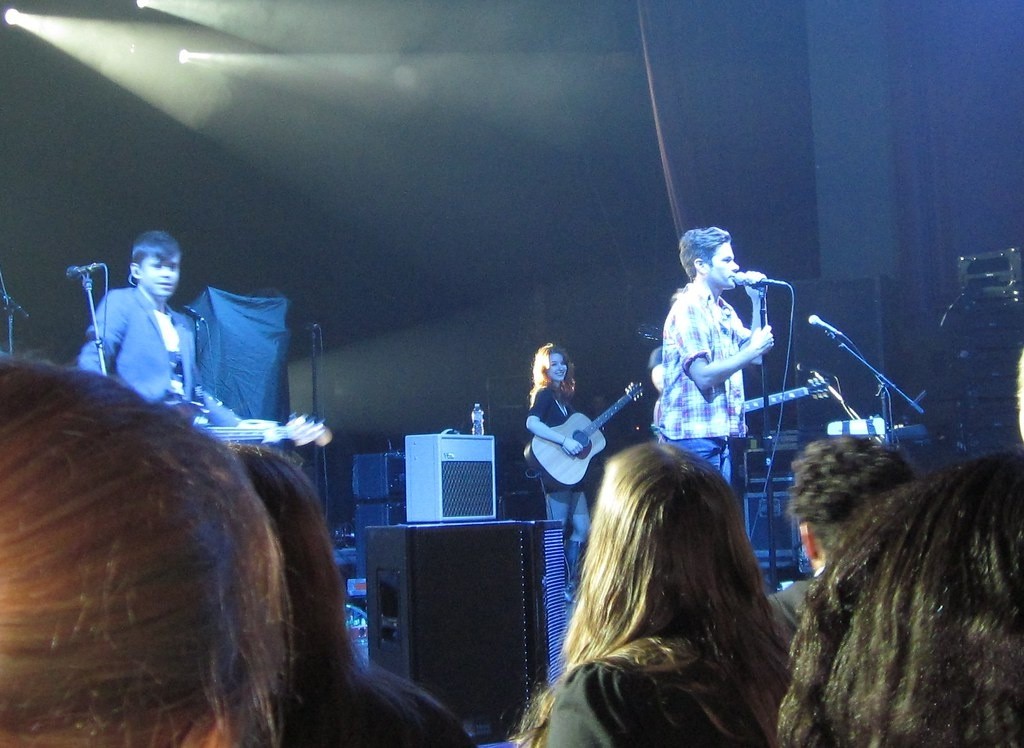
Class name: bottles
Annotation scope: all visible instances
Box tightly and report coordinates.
[471,403,484,435]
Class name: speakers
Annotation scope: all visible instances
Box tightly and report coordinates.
[955,244,1024,455]
[353,428,569,746]
[788,274,901,432]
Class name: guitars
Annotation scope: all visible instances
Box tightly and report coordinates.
[200,412,327,448]
[524,381,646,489]
[744,377,830,413]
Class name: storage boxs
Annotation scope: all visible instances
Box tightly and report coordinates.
[743,446,798,567]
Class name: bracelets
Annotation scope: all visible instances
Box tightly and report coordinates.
[560,437,566,444]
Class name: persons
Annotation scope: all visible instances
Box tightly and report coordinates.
[508,436,1023,748]
[647,347,665,394]
[1,350,474,746]
[77,229,280,443]
[659,227,773,493]
[525,341,591,601]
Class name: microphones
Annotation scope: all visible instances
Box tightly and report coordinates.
[182,305,207,323]
[809,315,843,335]
[67,263,105,282]
[735,272,788,288]
